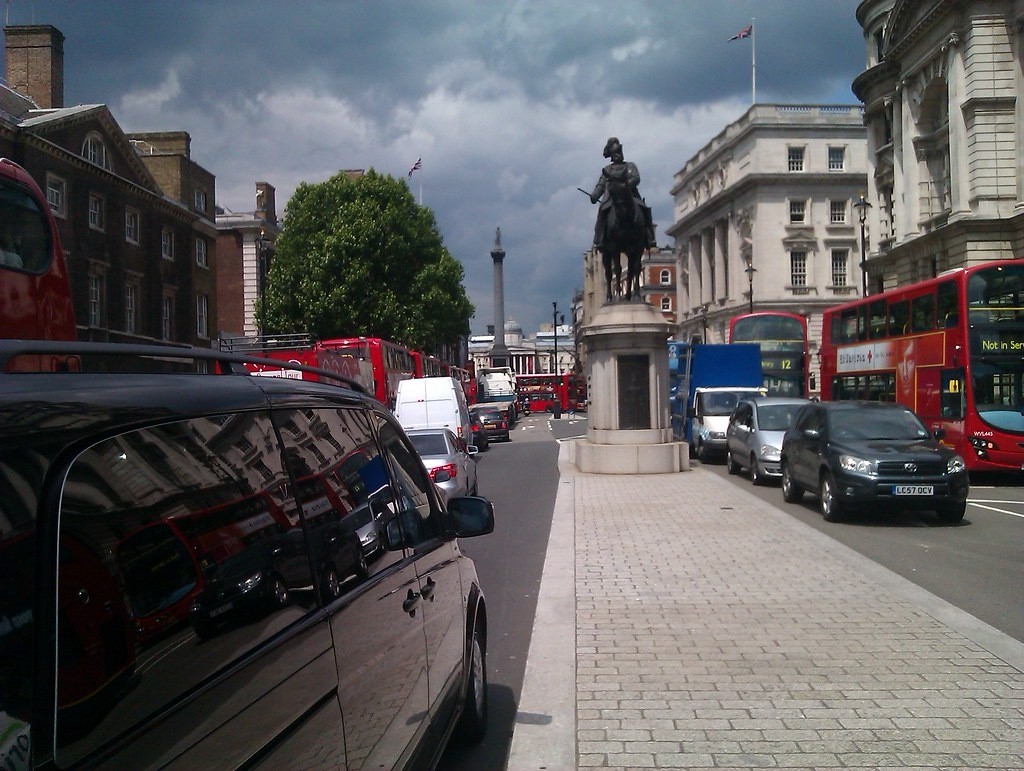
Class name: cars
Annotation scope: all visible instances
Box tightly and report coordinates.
[279,527,376,605]
[403,426,479,506]
[725,395,811,486]
[780,400,969,522]
[467,410,489,452]
[410,350,451,380]
[187,536,287,637]
[470,406,510,442]
[343,497,392,567]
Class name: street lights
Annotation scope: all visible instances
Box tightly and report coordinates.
[853,193,873,301]
[700,303,708,348]
[551,301,565,420]
[743,260,758,319]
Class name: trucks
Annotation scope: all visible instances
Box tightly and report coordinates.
[674,342,770,465]
[476,367,518,405]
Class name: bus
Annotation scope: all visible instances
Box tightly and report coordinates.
[818,257,1024,482]
[729,312,817,404]
[0,156,84,375]
[316,335,418,415]
[111,468,357,639]
[515,373,585,413]
[215,336,373,398]
[447,365,471,401]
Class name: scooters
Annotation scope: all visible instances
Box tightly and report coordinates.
[522,402,531,416]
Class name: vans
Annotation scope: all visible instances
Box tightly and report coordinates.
[393,375,474,455]
[1,337,493,771]
